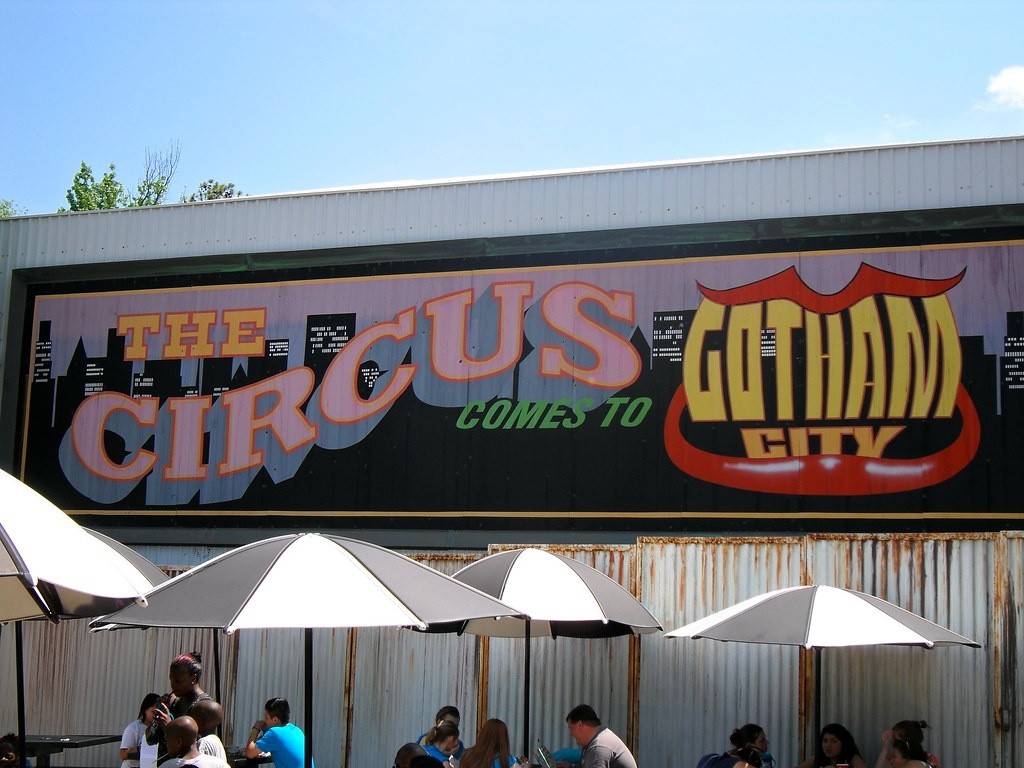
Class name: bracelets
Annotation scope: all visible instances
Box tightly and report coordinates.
[252,726,261,732]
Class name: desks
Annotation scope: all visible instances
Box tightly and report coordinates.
[24,735,122,768]
[228,757,273,768]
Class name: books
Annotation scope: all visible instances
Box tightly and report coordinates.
[535,746,557,768]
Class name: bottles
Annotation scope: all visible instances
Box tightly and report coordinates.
[145,694,172,745]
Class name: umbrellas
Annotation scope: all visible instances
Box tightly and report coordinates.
[663,585,981,768]
[398,548,664,763]
[89,532,531,768]
[0,469,174,768]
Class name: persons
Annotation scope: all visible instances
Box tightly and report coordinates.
[730,724,769,752]
[877,720,941,768]
[459,718,522,768]
[558,705,637,768]
[246,698,314,768]
[799,723,864,768]
[121,653,230,768]
[393,706,464,768]
